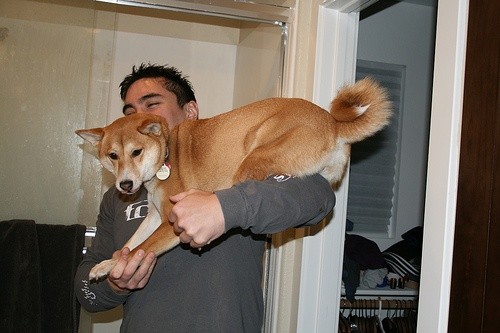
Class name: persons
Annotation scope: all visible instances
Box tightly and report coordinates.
[74,61,337,332]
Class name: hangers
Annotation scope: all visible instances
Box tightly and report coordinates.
[338,297,419,333]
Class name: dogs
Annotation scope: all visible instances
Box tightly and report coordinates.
[72,76,395,283]
